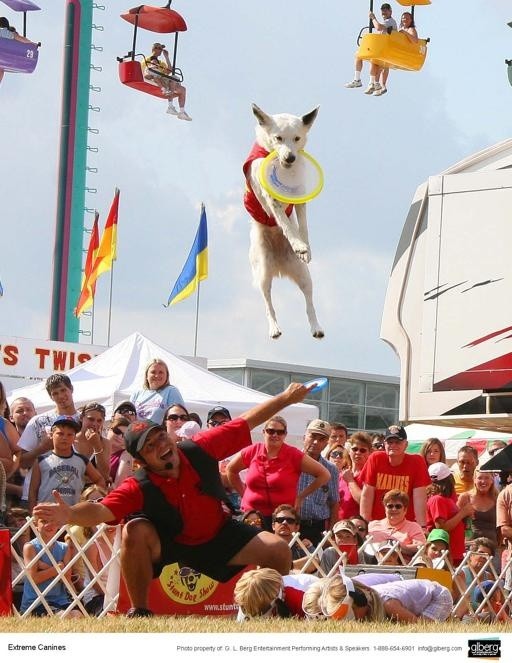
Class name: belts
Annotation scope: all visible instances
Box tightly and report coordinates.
[302,520,320,525]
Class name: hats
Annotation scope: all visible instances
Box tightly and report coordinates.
[302,573,356,620]
[207,406,231,422]
[378,540,400,552]
[307,419,331,437]
[427,529,449,545]
[385,425,407,440]
[174,421,200,438]
[237,588,284,623]
[428,462,454,481]
[474,580,495,602]
[332,520,359,536]
[124,418,167,454]
[114,400,135,412]
[51,416,81,434]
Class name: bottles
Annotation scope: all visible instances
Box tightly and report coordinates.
[464,516,472,538]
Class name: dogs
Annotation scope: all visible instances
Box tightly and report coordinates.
[242,102,325,339]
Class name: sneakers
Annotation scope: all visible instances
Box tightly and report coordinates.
[126,607,152,618]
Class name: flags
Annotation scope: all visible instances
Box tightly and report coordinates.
[86,195,120,299]
[161,210,209,307]
[74,219,100,318]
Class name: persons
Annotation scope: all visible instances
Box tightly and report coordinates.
[0,359,512,624]
[344,2,398,95]
[138,43,192,122]
[373,12,418,96]
[146,57,174,98]
[0,17,32,81]
[10,27,18,34]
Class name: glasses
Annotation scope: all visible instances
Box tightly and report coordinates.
[387,504,402,510]
[372,442,383,450]
[244,518,263,525]
[87,497,103,504]
[112,428,123,437]
[117,409,136,416]
[330,451,343,458]
[273,516,296,524]
[266,428,286,435]
[357,526,367,532]
[165,414,188,422]
[208,421,226,428]
[351,447,367,453]
